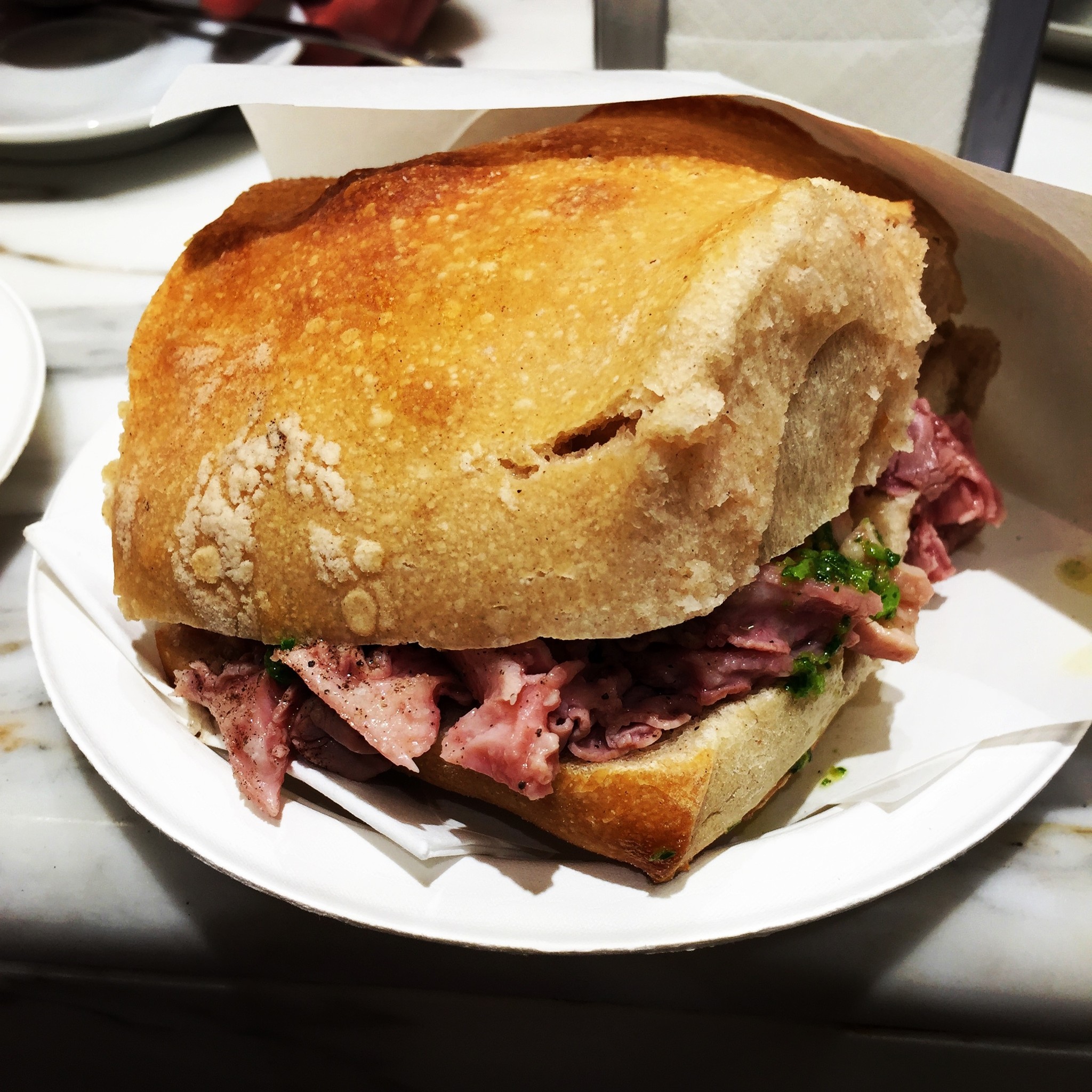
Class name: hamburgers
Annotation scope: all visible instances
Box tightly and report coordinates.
[100,95,1000,883]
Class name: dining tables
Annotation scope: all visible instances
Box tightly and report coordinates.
[0,0,1092,1092]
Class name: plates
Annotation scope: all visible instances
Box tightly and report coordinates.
[0,3,307,146]
[0,274,46,484]
[25,239,1092,955]
